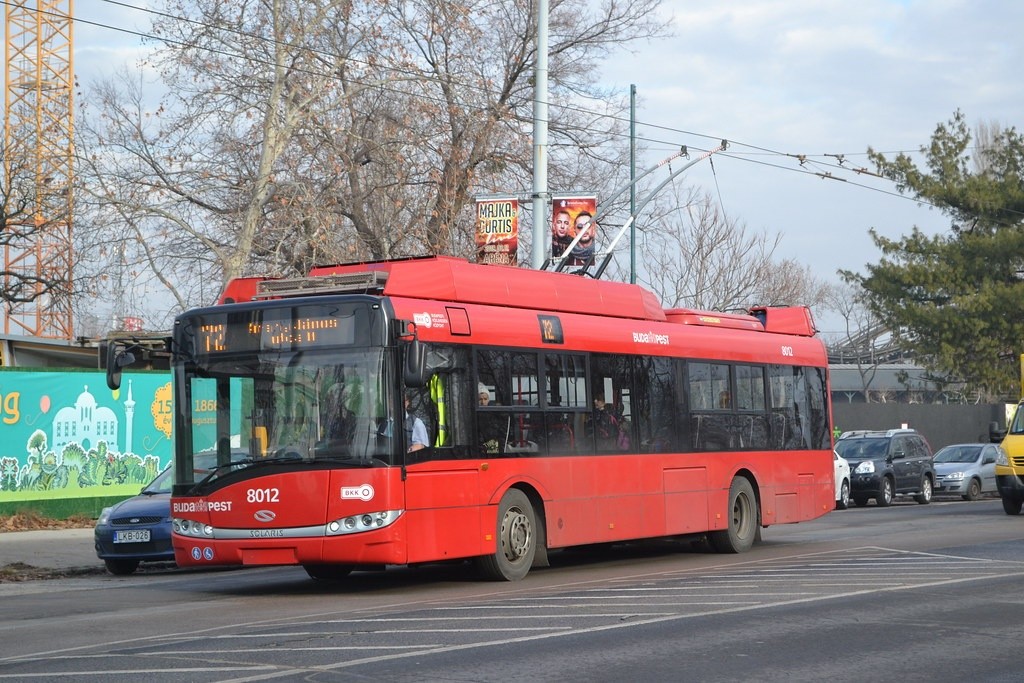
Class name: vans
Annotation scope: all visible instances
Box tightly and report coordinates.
[994,395,1024,515]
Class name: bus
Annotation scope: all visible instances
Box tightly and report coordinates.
[104,139,839,584]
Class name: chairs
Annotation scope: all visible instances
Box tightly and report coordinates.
[478,411,511,453]
[409,386,440,447]
[691,407,825,450]
[514,400,528,424]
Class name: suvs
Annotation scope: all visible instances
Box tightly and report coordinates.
[834,428,935,507]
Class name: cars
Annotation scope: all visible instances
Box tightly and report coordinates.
[832,450,852,509]
[94,446,252,576]
[931,442,1001,500]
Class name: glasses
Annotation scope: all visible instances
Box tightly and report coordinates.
[575,224,583,229]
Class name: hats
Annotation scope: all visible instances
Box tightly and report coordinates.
[477,382,490,399]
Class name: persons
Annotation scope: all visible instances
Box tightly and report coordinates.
[569,210,595,265]
[478,381,731,453]
[317,388,356,445]
[373,392,429,454]
[552,210,574,266]
